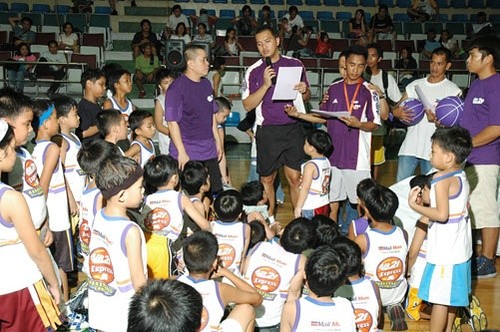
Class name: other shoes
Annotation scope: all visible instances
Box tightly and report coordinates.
[25,74,37,81]
[386,303,408,330]
[139,91,145,98]
[47,89,55,97]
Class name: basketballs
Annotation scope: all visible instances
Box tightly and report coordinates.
[435,95,467,128]
[402,101,426,126]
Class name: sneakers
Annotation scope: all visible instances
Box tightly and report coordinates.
[451,308,473,332]
[465,295,488,332]
[472,255,496,278]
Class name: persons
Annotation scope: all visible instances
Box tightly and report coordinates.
[407,0,438,22]
[9,14,80,98]
[130,5,500,60]
[69,0,137,17]
[0,25,500,332]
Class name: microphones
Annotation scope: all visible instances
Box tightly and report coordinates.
[266,57,276,84]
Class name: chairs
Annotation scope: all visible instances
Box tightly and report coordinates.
[182,0,500,96]
[224,111,240,127]
[0,1,112,94]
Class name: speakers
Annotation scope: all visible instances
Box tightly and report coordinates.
[165,39,186,70]
[191,42,212,67]
